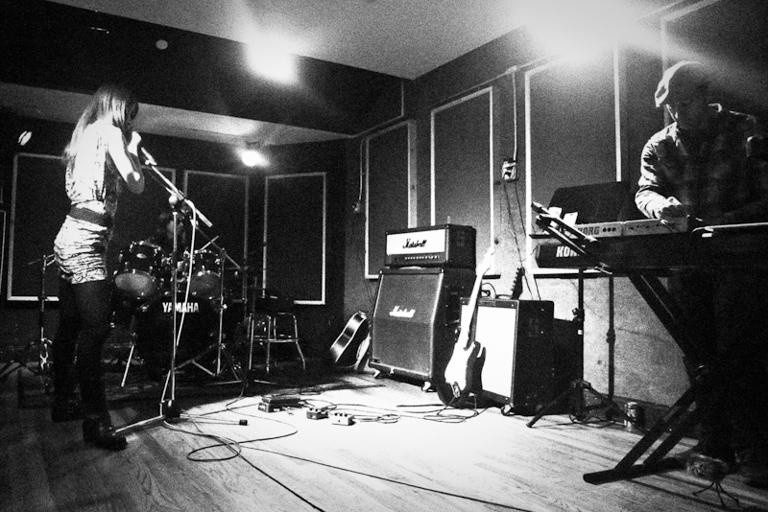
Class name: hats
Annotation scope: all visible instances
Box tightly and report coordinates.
[655,60,706,108]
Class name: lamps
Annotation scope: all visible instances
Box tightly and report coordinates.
[16,129,33,146]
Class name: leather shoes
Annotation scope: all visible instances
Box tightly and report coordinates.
[84,414,125,451]
[53,394,80,424]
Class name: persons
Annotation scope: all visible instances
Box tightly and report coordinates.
[137,204,213,380]
[634,62,768,488]
[49,82,145,450]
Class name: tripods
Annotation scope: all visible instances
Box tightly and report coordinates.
[170,220,245,386]
[114,146,247,436]
[0,256,54,392]
[527,269,636,427]
[203,277,299,394]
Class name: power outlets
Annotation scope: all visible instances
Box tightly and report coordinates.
[351,202,361,214]
[501,162,517,182]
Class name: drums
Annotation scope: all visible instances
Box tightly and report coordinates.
[132,293,213,372]
[114,240,165,298]
[180,251,221,295]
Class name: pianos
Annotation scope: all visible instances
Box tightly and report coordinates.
[531,217,764,280]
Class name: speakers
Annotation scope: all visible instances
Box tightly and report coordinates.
[369,266,476,392]
[459,296,554,414]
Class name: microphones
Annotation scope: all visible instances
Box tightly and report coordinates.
[584,235,600,248]
[126,128,144,151]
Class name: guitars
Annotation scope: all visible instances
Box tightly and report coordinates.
[330,310,370,367]
[438,245,495,391]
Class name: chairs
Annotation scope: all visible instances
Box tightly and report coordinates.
[249,309,307,371]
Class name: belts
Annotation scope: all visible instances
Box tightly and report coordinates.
[68,207,111,227]
